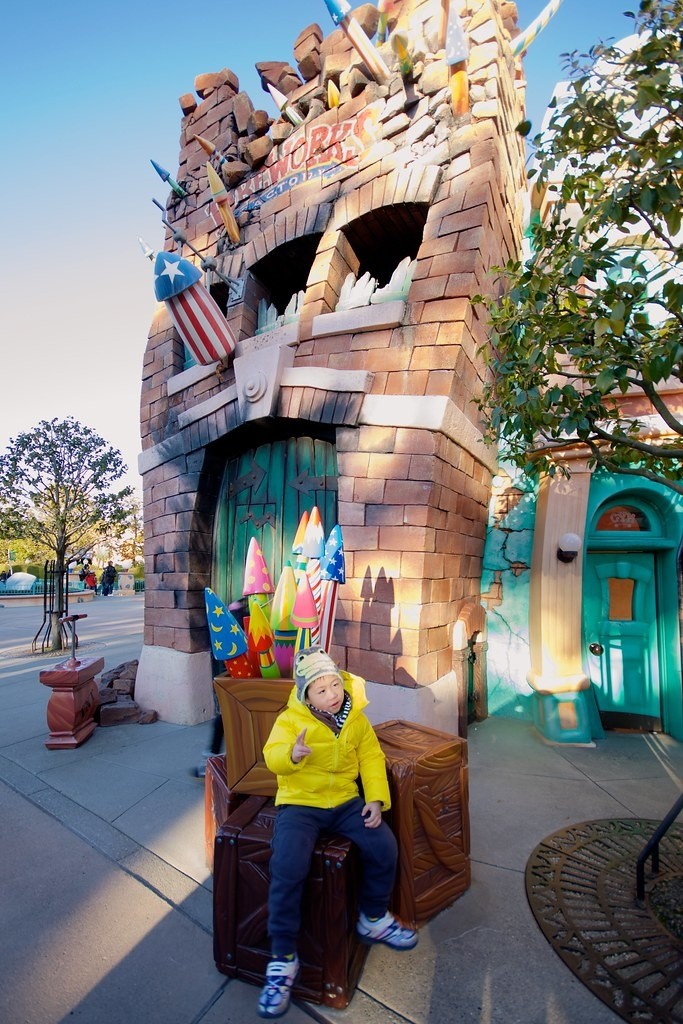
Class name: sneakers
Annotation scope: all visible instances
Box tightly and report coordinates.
[257,953,300,1018]
[356,912,418,951]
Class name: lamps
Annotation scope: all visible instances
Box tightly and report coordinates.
[556,533,582,563]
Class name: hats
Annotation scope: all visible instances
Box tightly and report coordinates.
[293,646,343,702]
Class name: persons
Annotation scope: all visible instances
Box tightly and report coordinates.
[0,570,6,582]
[78,560,116,596]
[6,569,11,578]
[256,644,419,1019]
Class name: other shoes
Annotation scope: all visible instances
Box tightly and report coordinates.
[191,751,223,781]
